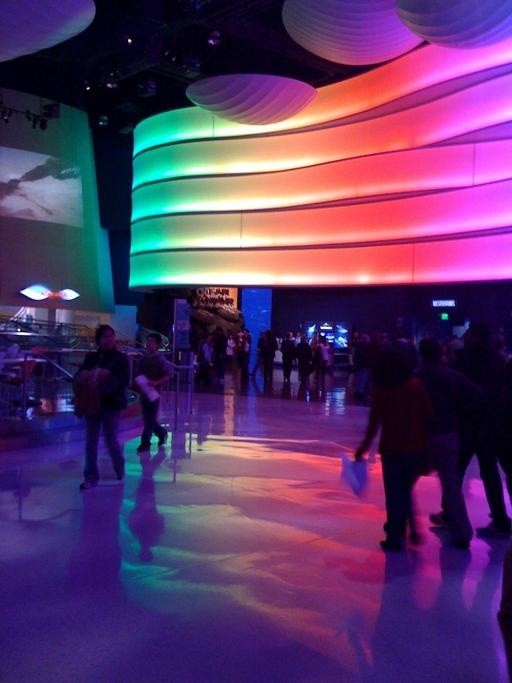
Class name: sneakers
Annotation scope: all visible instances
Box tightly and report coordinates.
[476,519,512,538]
[158,428,167,446]
[429,512,446,524]
[138,442,150,451]
[429,527,470,548]
[380,539,406,551]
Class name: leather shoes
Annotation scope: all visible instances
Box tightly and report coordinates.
[113,460,124,479]
[80,476,98,488]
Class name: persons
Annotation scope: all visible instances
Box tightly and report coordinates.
[132,333,173,452]
[203,326,329,386]
[73,324,130,489]
[348,324,512,553]
